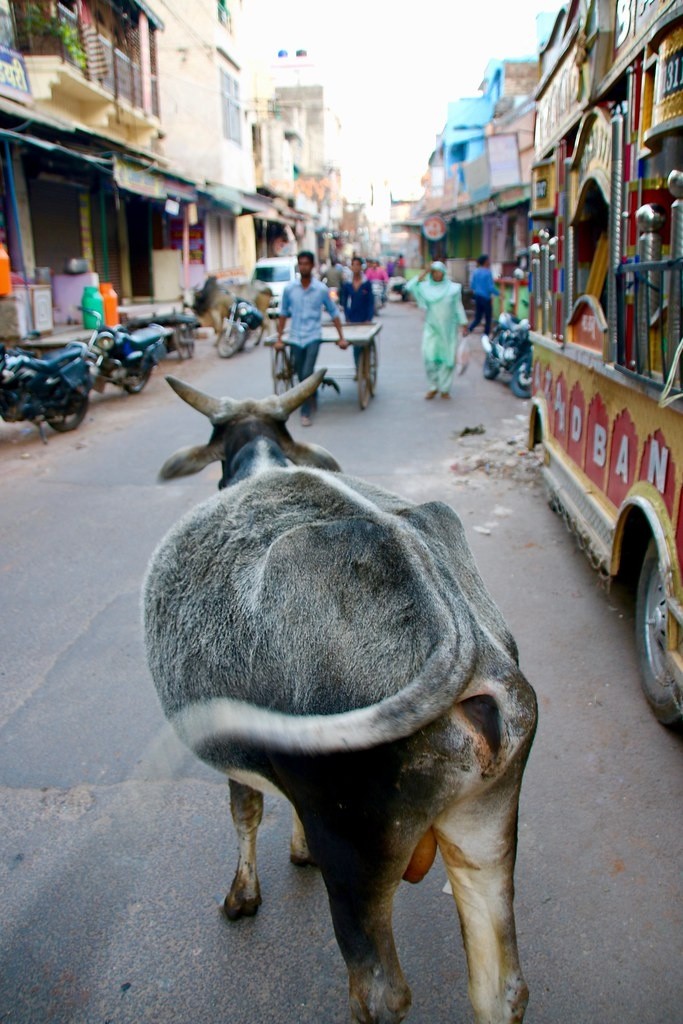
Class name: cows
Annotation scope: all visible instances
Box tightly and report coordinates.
[0,365,540,1023]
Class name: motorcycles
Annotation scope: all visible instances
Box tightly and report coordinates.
[0,328,97,446]
[66,305,177,396]
[217,288,266,360]
[483,310,535,399]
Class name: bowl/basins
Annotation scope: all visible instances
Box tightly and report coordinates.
[65,258,89,274]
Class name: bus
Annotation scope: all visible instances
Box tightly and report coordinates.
[521,0,683,732]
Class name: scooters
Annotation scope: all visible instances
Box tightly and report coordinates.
[321,287,345,313]
[368,276,389,312]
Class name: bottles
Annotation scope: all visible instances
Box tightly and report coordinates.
[100,282,119,326]
[0,243,11,297]
[81,286,105,329]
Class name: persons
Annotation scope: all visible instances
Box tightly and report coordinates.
[275,251,348,427]
[320,254,408,316]
[405,261,468,400]
[340,258,375,381]
[467,254,502,338]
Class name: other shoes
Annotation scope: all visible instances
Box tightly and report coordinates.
[299,415,312,426]
[462,326,469,338]
[425,388,439,399]
[441,391,451,400]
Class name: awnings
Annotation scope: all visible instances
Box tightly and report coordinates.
[394,182,532,231]
[1,86,309,228]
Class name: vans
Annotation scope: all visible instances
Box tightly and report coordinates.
[247,255,298,319]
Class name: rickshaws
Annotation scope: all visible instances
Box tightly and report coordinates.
[263,319,383,411]
